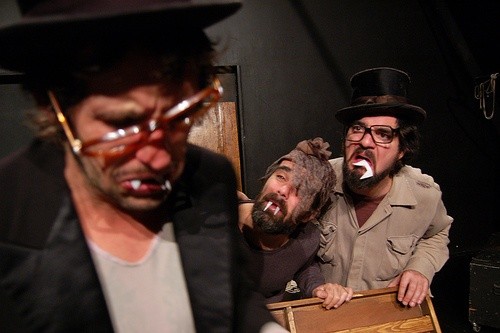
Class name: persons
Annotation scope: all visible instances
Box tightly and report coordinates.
[239,138,352,309]
[0,1,290,333]
[238,68,454,307]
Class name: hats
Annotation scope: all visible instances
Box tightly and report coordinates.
[258,136,337,211]
[334,67,426,127]
[0,0,243,74]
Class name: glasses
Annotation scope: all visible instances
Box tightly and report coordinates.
[344,122,415,144]
[46,74,224,160]
